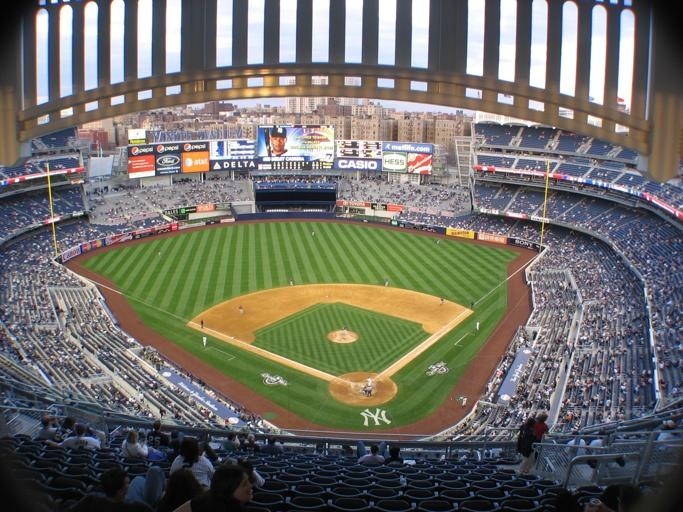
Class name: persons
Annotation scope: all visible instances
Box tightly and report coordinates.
[120,176,510,242]
[435,350,509,443]
[119,331,289,512]
[365,376,372,387]
[361,386,367,395]
[512,123,680,511]
[365,385,372,397]
[0,163,121,512]
[356,439,387,466]
[312,442,328,456]
[260,126,295,157]
[383,444,404,465]
[338,443,356,466]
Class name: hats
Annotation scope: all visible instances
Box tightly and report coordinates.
[662,420,675,429]
[270,128,287,138]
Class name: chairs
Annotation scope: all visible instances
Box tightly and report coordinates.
[2,414,614,511]
